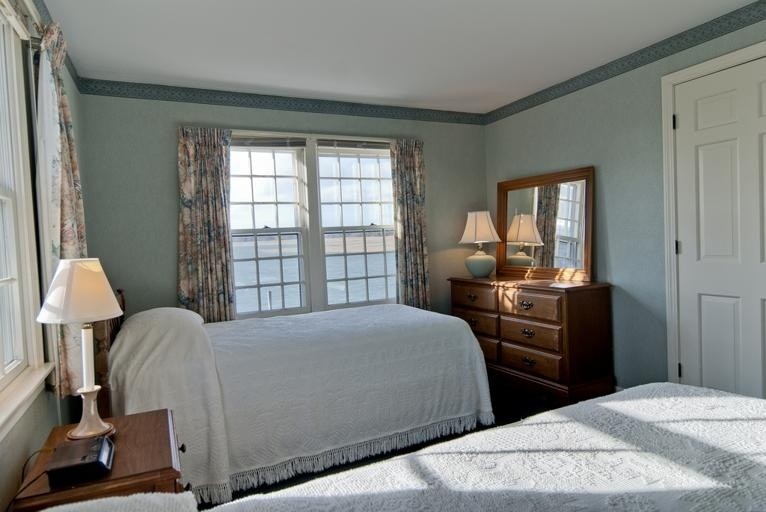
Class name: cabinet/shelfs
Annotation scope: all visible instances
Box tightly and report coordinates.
[446,275,616,408]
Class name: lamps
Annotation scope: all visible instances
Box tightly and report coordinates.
[457,210,503,278]
[507,214,545,267]
[36,257,125,443]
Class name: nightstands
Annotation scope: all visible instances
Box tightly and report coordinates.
[6,408,193,512]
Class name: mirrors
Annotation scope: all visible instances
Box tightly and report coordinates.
[495,165,595,282]
